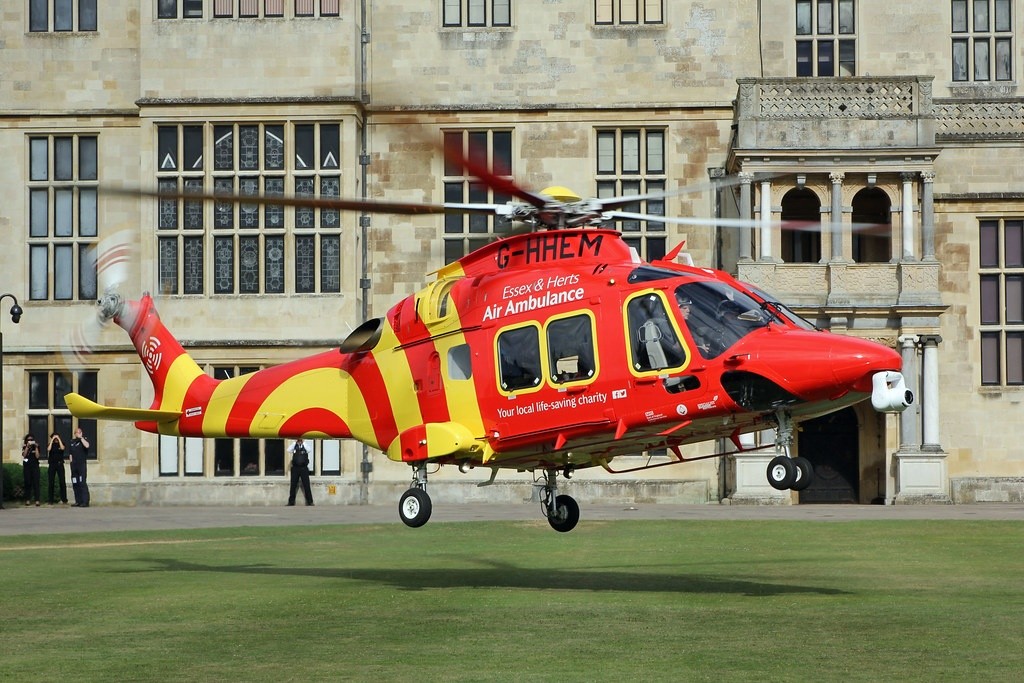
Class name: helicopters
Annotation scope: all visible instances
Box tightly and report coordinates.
[63,103,914,533]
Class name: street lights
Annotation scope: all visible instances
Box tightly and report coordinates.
[0,293,23,509]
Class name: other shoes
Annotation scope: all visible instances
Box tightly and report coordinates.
[35,501,40,506]
[62,498,68,502]
[306,503,314,506]
[79,503,89,507]
[48,500,53,505]
[286,501,294,505]
[25,500,31,505]
[71,502,81,506]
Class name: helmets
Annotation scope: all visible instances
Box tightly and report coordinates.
[716,300,741,320]
[674,293,693,308]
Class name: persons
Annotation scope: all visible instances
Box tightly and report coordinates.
[68,429,91,507]
[22,434,41,506]
[658,293,739,366]
[45,432,68,506]
[285,438,314,506]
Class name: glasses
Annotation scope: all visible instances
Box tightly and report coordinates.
[26,439,33,441]
